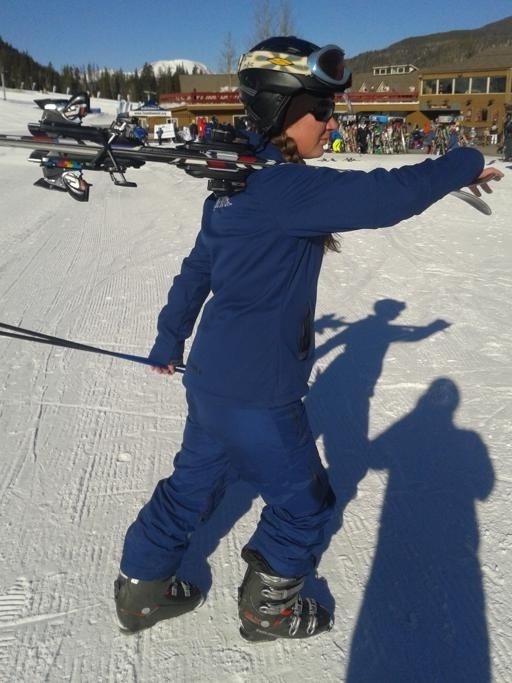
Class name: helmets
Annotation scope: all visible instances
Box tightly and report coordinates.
[238,36,353,136]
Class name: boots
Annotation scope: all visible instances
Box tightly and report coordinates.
[114,570,204,635]
[238,564,335,644]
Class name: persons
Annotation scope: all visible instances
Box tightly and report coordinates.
[114,35,506,644]
[326,116,499,155]
[505,104,512,161]
[187,115,218,141]
[133,123,150,142]
[157,128,163,145]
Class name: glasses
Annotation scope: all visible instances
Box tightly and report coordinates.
[302,99,336,122]
[237,44,353,92]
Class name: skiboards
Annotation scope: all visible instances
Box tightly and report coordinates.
[1,133,493,217]
